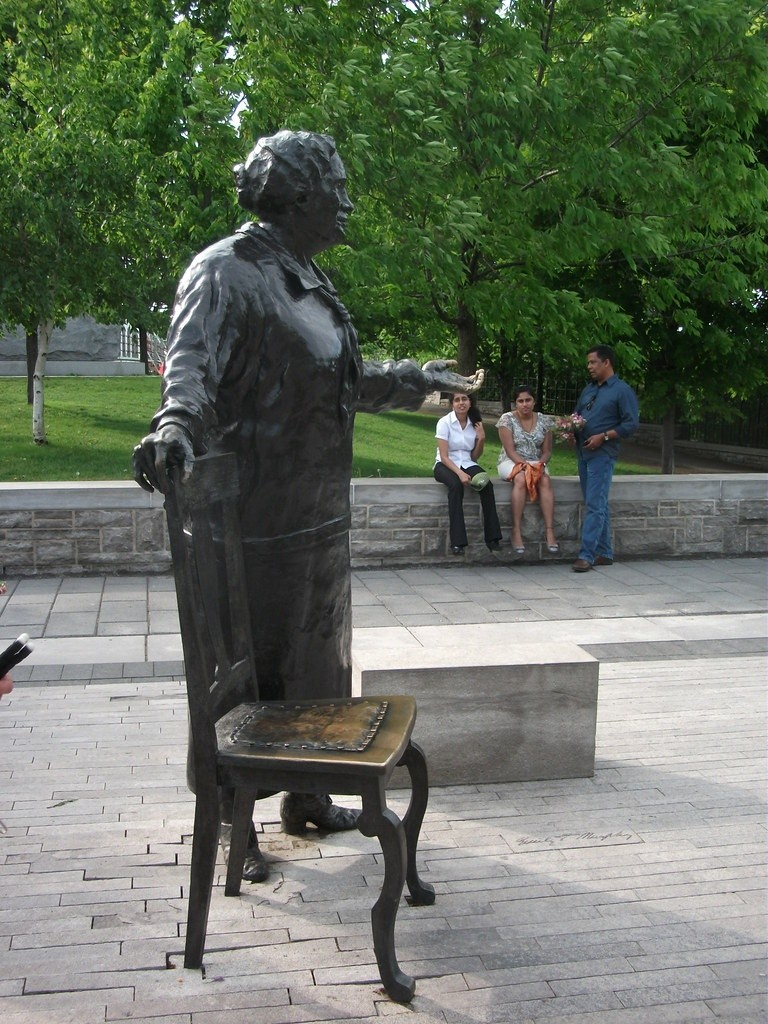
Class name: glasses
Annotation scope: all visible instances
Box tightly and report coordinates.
[587,394,596,410]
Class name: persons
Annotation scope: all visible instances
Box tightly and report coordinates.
[0,671,14,702]
[495,386,559,553]
[432,391,503,557]
[131,129,485,883]
[566,345,639,572]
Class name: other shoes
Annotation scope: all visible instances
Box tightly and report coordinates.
[572,559,591,572]
[510,537,525,553]
[593,556,612,565]
[545,537,558,552]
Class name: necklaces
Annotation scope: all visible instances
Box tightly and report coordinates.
[515,411,534,434]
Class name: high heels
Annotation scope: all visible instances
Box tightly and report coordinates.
[451,545,465,555]
[486,540,503,552]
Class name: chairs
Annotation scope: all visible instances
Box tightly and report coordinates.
[162,452,437,1005]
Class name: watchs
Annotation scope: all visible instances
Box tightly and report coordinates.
[537,461,546,467]
[603,431,609,441]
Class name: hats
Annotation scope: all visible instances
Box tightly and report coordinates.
[470,472,489,492]
